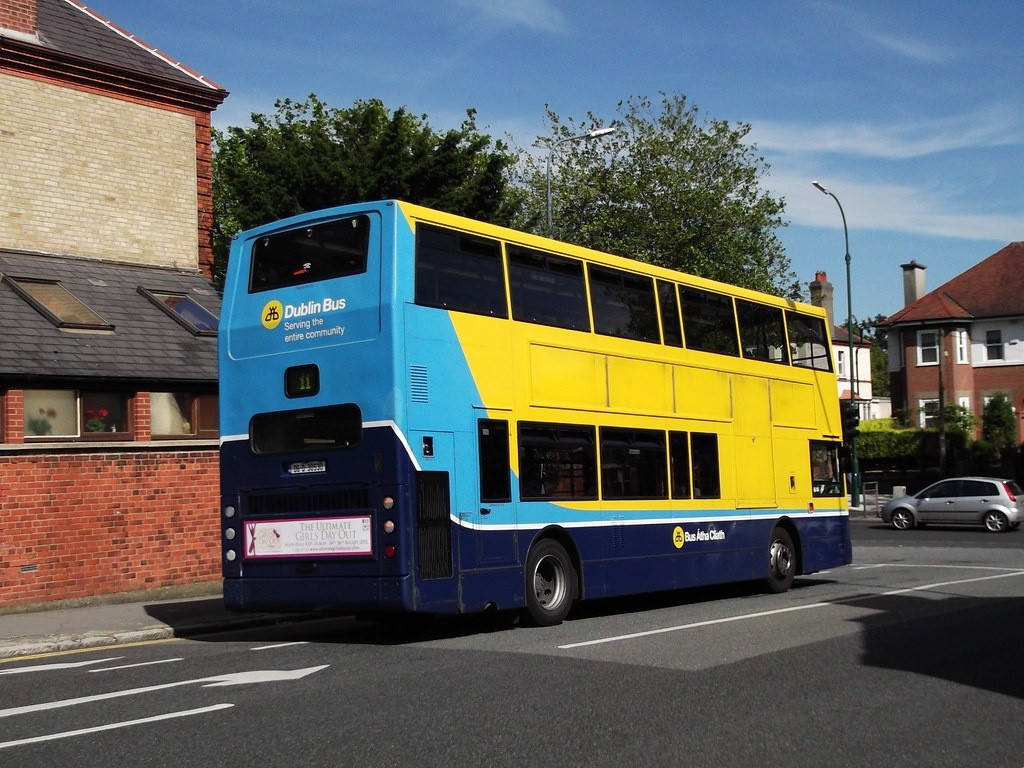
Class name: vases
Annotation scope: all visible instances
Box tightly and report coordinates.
[37,432,46,435]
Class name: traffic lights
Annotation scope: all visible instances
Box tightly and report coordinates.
[839,399,860,443]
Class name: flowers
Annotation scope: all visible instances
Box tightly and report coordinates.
[82,407,109,432]
[28,408,57,436]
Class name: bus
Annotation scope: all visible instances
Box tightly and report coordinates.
[221,196,854,628]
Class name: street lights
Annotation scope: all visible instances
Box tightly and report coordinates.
[812,178,860,507]
[546,127,616,237]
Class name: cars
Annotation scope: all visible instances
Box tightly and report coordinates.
[881,476,1024,533]
